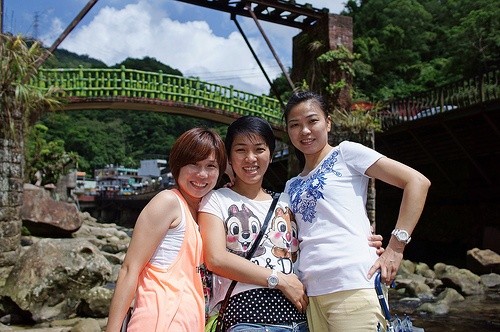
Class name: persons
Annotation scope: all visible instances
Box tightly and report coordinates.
[198,116,385,332]
[280,90,432,332]
[106,128,228,332]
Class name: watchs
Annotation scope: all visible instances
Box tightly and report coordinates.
[266,270,280,289]
[391,229,411,244]
